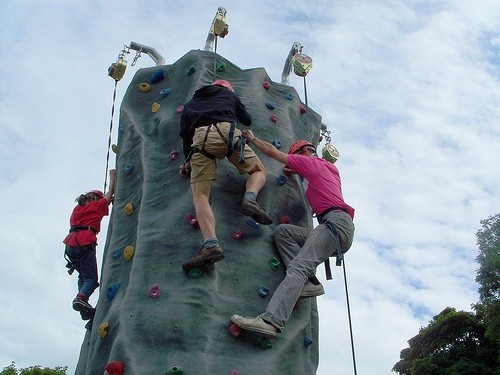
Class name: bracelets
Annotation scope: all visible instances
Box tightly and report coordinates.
[250,136,258,142]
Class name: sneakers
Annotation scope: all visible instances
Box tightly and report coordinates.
[80,311,90,320]
[230,314,276,336]
[241,197,273,225]
[300,281,325,296]
[182,244,224,270]
[73,298,96,314]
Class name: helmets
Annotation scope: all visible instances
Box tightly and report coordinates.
[212,79,234,91]
[87,190,103,197]
[288,140,316,155]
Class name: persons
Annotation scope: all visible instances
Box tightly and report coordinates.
[230,129,355,337]
[178,80,273,273]
[62,169,116,321]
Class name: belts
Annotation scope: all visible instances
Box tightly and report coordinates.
[317,207,347,218]
